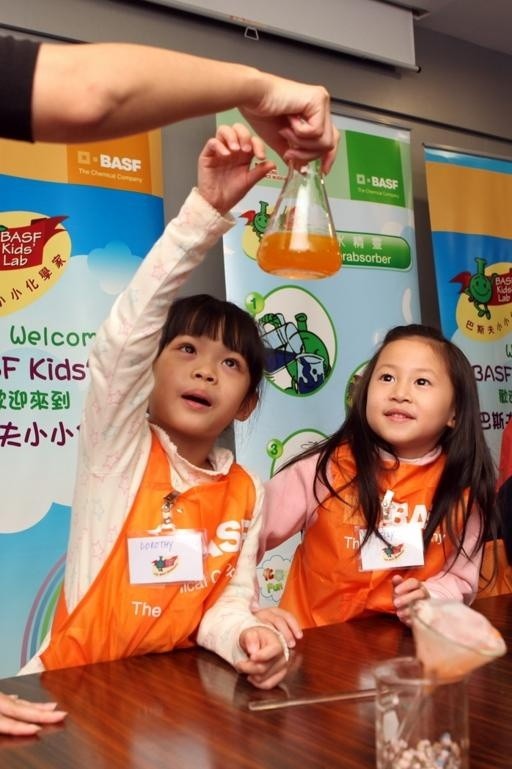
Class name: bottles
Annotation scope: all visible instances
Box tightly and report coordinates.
[257,156,340,280]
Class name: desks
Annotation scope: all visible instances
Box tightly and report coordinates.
[0,592,511,769]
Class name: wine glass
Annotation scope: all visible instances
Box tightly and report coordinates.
[385,596,508,764]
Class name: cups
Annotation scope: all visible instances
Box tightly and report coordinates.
[376,657,469,769]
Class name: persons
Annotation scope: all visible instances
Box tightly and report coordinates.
[0,34,342,740]
[14,121,292,693]
[256,323,503,631]
